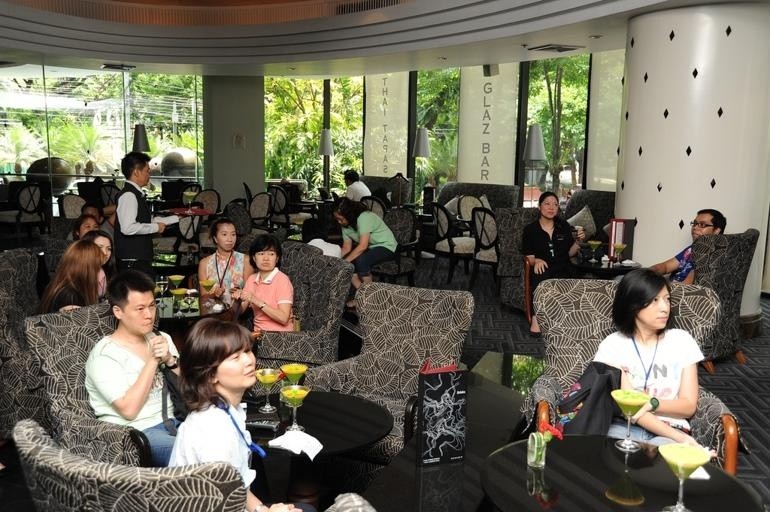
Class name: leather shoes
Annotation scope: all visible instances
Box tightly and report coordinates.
[531,317,542,338]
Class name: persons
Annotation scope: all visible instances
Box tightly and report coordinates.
[647,208,727,287]
[343,169,372,203]
[592,267,707,446]
[37,200,118,314]
[168,317,305,511]
[83,270,183,467]
[231,235,294,331]
[197,219,254,322]
[521,191,586,338]
[113,151,191,284]
[331,197,399,309]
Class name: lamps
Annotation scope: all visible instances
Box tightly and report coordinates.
[132,124,150,152]
[522,125,546,208]
[412,127,431,157]
[318,129,334,199]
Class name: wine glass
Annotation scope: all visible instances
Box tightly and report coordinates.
[183,191,197,213]
[613,244,627,266]
[610,389,649,454]
[255,364,312,432]
[658,443,712,511]
[587,241,601,263]
[156,275,216,316]
[605,452,644,505]
[571,231,581,250]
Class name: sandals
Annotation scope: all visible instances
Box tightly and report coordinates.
[345,300,356,310]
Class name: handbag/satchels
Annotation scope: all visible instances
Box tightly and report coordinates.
[159,360,189,435]
[553,360,621,437]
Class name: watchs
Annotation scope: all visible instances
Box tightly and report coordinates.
[650,398,661,412]
[166,355,179,370]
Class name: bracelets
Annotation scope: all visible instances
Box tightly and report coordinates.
[252,503,265,512]
[259,302,267,310]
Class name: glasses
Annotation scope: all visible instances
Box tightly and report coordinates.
[690,221,714,228]
[146,167,150,174]
[548,240,555,257]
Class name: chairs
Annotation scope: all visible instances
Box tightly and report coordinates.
[0,248,38,438]
[610,228,760,376]
[12,418,247,512]
[533,278,737,475]
[24,301,159,467]
[303,282,475,479]
[246,255,354,397]
